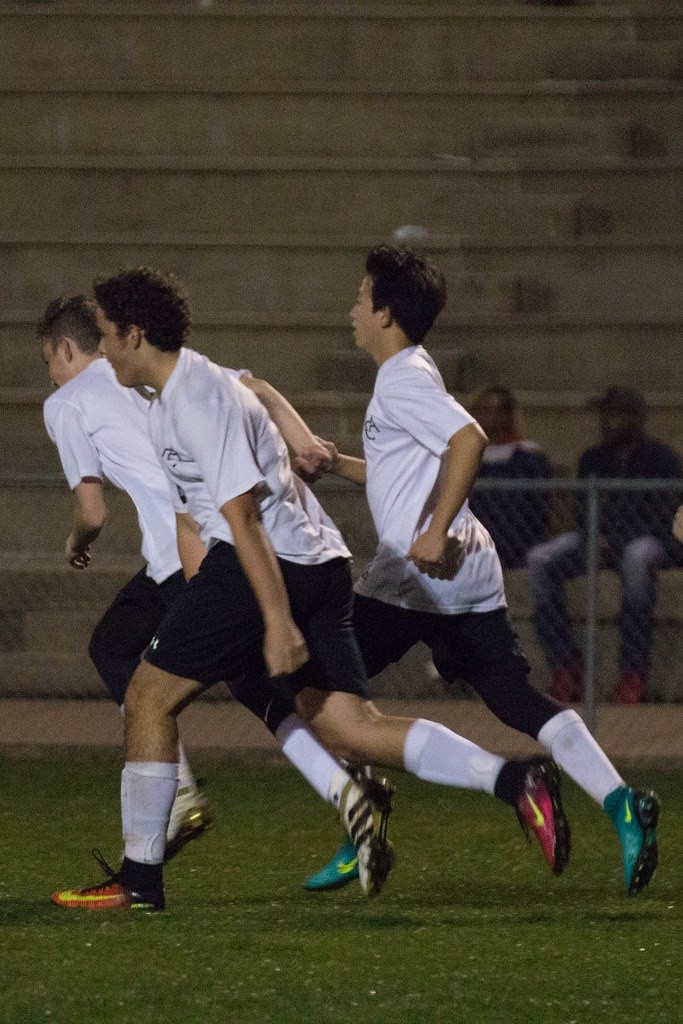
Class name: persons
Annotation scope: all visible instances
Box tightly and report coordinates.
[38,294,394,893]
[526,384,682,708]
[461,385,550,568]
[50,268,576,908]
[299,242,665,894]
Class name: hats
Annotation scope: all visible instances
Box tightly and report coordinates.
[590,387,646,414]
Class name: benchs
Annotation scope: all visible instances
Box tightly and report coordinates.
[0,0,683,690]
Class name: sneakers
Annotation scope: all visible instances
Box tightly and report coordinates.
[612,786,661,894]
[49,848,165,912]
[514,754,570,876]
[164,785,206,861]
[301,842,360,890]
[340,775,392,898]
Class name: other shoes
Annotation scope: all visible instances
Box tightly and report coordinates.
[547,669,576,701]
[616,669,644,704]
